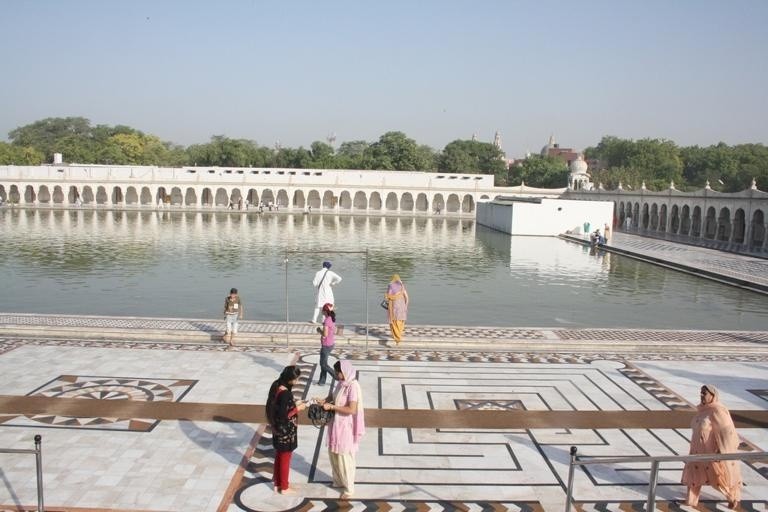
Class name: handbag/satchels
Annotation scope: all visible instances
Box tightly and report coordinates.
[308,404,333,421]
[381,300,388,310]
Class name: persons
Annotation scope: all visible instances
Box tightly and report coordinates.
[221,288,243,336]
[676,385,742,509]
[384,274,408,343]
[311,262,342,323]
[265,366,309,495]
[583,215,632,247]
[318,360,364,500]
[317,303,336,386]
